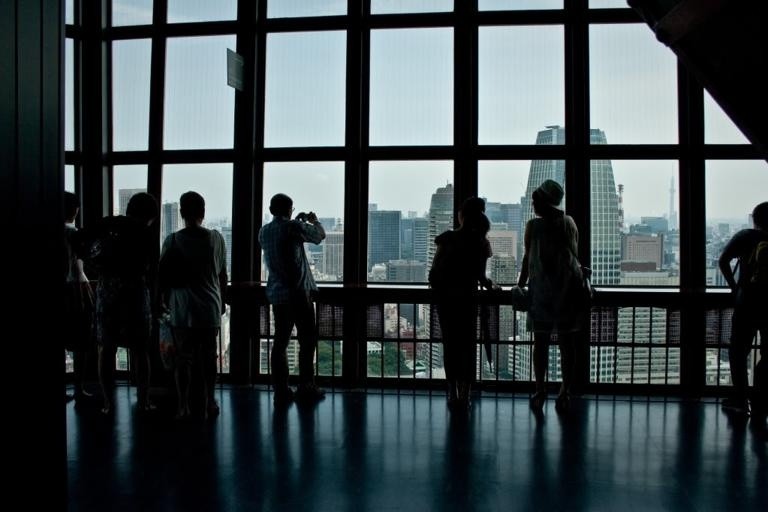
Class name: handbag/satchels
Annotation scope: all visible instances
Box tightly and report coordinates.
[155,308,195,371]
[572,250,591,278]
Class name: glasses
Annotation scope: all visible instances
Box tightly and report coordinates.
[292,207,295,213]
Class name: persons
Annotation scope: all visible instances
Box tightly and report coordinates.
[427,196,494,416]
[720,201,768,414]
[62,191,100,410]
[259,195,328,400]
[157,193,227,424]
[73,195,162,414]
[511,178,595,410]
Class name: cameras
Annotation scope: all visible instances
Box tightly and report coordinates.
[300,212,312,221]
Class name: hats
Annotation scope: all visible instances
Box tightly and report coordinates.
[540,179,564,201]
